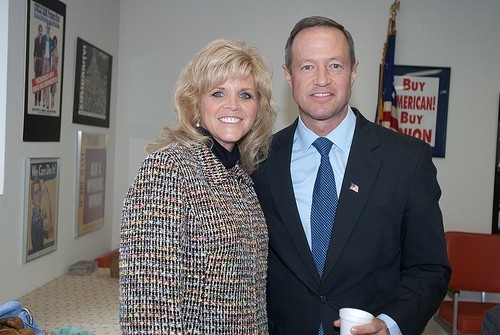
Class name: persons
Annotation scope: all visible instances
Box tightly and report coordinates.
[251,16,452,335]
[119,40,278,335]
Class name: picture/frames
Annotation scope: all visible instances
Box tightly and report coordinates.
[22,157,61,263]
[374,63,451,157]
[74,131,109,238]
[72,37,113,128]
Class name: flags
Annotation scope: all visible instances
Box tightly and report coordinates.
[374,0,400,135]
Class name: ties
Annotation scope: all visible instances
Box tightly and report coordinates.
[310,137,339,335]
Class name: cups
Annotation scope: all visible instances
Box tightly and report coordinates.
[338,308,374,335]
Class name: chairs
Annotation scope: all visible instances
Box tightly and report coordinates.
[434,232,500,335]
